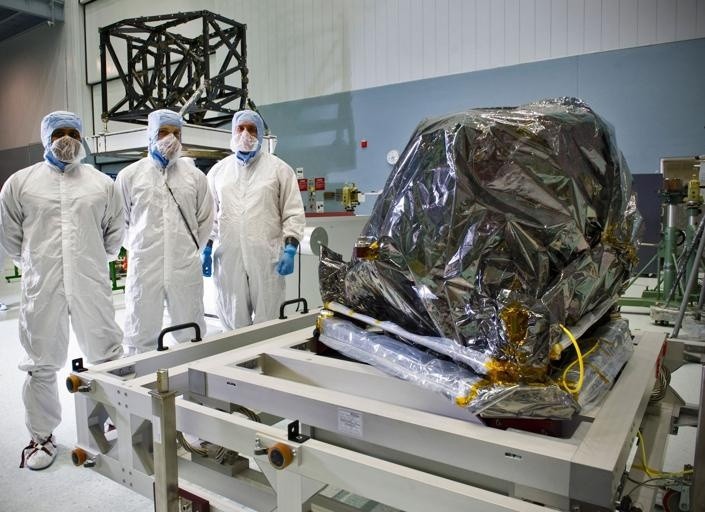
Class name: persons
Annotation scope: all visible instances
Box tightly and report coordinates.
[1,110,124,470]
[117,109,215,354]
[202,109,307,334]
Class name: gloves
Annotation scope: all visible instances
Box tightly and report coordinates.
[278,244,296,275]
[202,246,212,277]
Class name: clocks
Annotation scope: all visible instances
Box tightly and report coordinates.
[387,150,399,165]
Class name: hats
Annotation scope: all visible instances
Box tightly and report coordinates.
[148,109,182,146]
[40,111,83,153]
[232,110,264,145]
